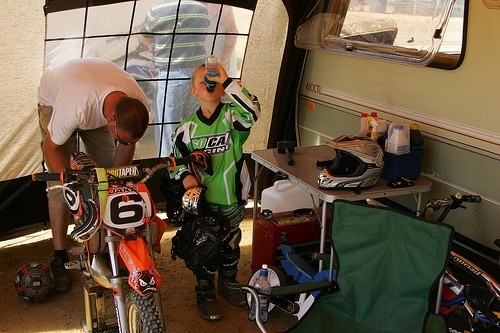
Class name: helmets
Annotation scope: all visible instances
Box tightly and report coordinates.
[317,134,385,191]
[181,185,214,216]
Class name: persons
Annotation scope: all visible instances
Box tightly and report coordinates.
[137,0,212,157]
[167,60,262,321]
[201,1,240,77]
[37,57,154,294]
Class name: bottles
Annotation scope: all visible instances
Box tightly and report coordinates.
[442,268,465,296]
[260,179,320,213]
[248,264,272,322]
[203,55,219,93]
[359,111,386,140]
[386,125,410,155]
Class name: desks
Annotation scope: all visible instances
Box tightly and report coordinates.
[250,145,432,292]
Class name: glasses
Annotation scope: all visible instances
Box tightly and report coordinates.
[114,137,140,147]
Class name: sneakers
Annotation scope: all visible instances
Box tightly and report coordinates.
[50,255,71,292]
[217,269,247,305]
[195,269,221,320]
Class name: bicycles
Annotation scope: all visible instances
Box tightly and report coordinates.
[31,153,199,333]
[422,193,500,332]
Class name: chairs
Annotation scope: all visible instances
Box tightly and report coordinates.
[240,199,455,333]
